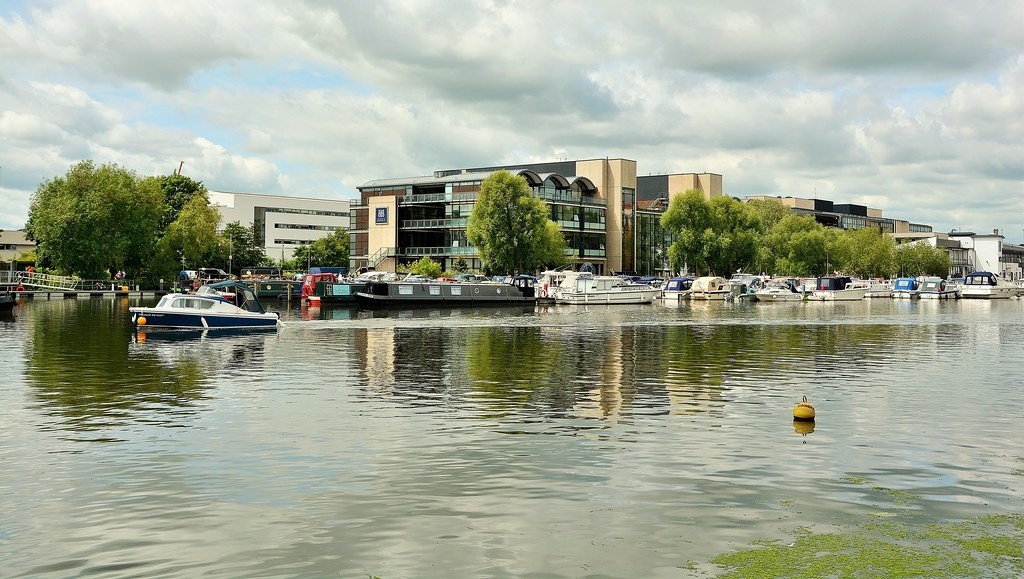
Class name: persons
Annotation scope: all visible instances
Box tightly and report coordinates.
[610,267,615,276]
[761,271,767,282]
[115,268,126,280]
[25,266,34,276]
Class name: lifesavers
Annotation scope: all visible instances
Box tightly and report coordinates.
[16,287,26,292]
[540,290,548,298]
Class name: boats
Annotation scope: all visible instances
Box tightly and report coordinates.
[352,273,537,305]
[0,290,18,309]
[355,260,1024,305]
[129,266,347,330]
